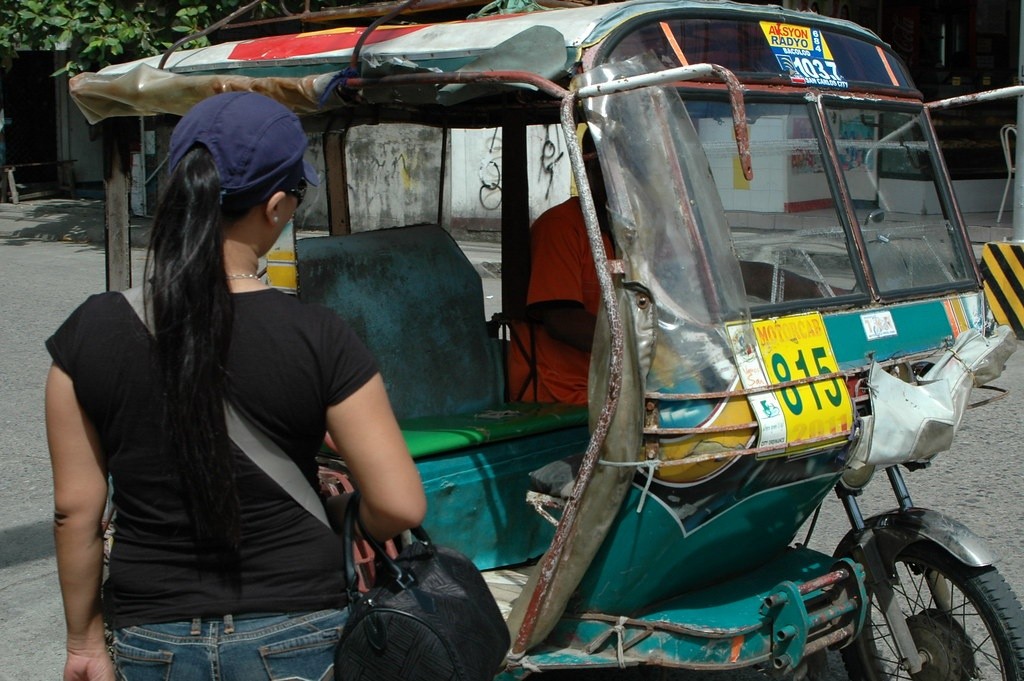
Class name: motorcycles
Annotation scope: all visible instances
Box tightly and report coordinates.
[71,0,1023,681]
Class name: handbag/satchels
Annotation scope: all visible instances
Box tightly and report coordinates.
[332,488,512,681]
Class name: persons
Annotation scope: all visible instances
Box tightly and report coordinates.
[44,91,427,681]
[508,126,619,405]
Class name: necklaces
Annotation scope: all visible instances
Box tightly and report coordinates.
[225,273,261,283]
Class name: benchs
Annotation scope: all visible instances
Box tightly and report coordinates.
[294,223,591,573]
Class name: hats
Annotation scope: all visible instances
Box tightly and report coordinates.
[167,89,319,197]
